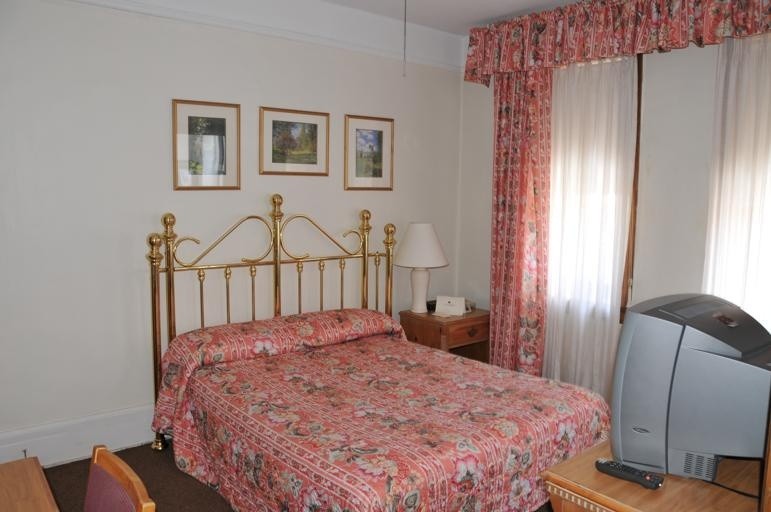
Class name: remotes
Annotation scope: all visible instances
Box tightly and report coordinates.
[595,458,664,490]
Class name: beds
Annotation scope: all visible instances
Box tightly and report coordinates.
[147,193,611,511]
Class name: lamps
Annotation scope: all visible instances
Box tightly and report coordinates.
[394,221,450,314]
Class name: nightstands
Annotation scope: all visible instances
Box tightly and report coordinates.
[399,307,490,365]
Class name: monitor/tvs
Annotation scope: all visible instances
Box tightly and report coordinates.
[610,292,771,482]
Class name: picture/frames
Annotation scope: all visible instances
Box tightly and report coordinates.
[173,98,395,191]
